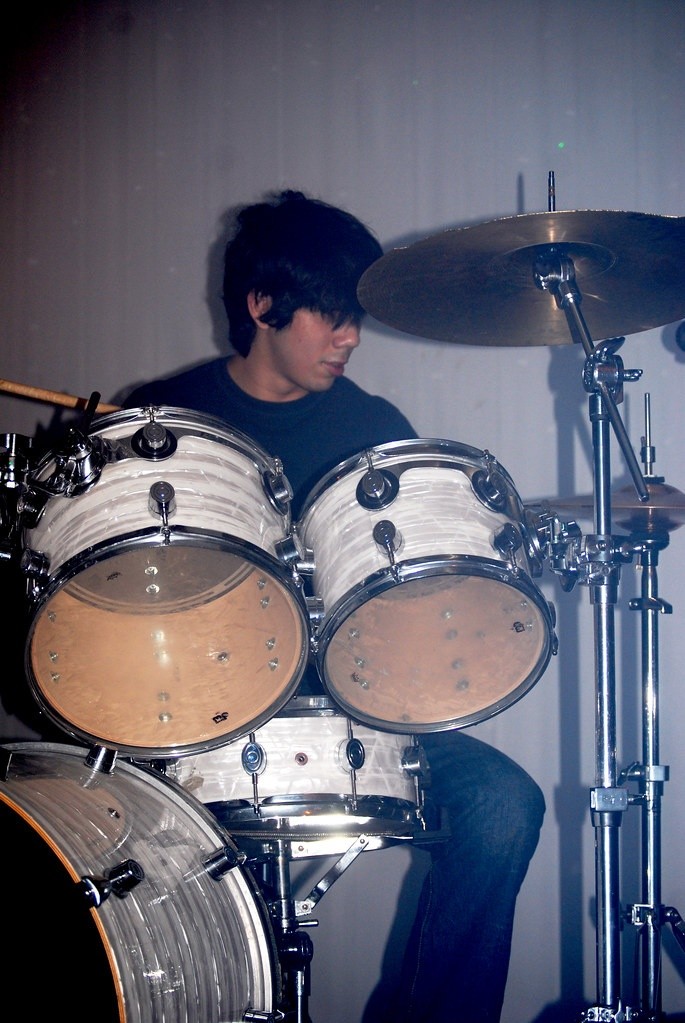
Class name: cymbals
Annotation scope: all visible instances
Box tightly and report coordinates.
[355,209,684,347]
[520,478,685,535]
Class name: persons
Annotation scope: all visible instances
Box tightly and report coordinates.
[117,187,548,1023]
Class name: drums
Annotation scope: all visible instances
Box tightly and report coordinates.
[291,435,556,736]
[9,403,313,764]
[1,738,284,1023]
[124,693,429,865]
